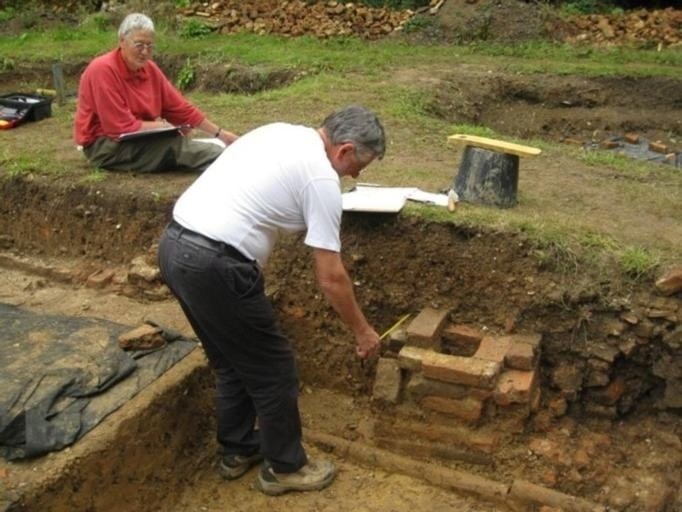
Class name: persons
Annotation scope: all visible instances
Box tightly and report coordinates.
[156,106,388,496]
[73,12,240,175]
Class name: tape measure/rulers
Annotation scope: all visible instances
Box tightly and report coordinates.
[360,312,413,367]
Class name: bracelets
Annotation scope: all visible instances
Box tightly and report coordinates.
[215,127,222,138]
[163,119,167,128]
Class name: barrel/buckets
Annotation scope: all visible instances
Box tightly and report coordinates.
[450,145,519,209]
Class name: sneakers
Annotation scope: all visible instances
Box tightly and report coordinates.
[220,455,335,496]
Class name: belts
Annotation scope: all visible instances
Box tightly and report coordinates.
[168,221,248,262]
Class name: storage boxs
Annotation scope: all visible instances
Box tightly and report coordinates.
[0,89,56,130]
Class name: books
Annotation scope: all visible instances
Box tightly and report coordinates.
[120,126,176,140]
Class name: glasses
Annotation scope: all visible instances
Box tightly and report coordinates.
[127,39,153,48]
